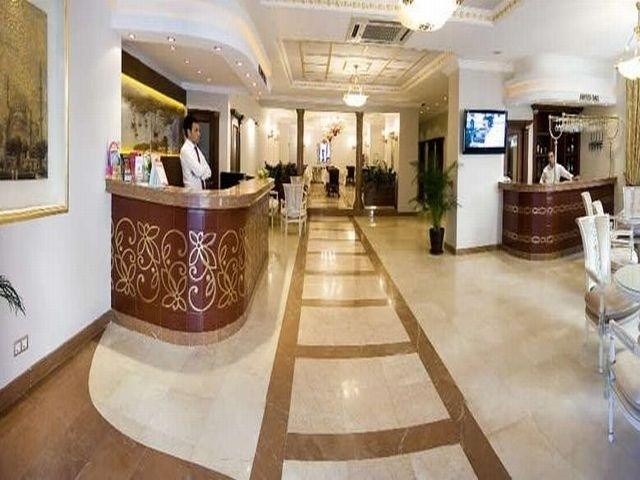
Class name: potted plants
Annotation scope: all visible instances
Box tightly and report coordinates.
[407,159,459,255]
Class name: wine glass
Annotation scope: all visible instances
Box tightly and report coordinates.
[554,116,603,134]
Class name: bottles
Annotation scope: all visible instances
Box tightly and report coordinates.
[535,143,547,173]
[566,137,575,173]
[111,150,152,183]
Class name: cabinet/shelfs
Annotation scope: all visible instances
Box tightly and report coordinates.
[532,111,582,184]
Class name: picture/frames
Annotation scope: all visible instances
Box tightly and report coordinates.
[0,0,71,226]
[614,1,640,80]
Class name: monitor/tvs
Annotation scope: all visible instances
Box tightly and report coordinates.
[462,109,508,154]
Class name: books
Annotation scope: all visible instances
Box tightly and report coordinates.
[106,137,169,189]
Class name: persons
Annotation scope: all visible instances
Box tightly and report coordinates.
[179,114,213,191]
[482,116,504,145]
[539,149,581,185]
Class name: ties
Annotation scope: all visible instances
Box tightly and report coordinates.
[194,146,204,189]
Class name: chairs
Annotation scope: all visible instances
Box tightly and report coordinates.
[268,175,307,237]
[575,185,640,448]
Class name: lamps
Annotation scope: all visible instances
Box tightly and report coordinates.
[342,64,370,108]
